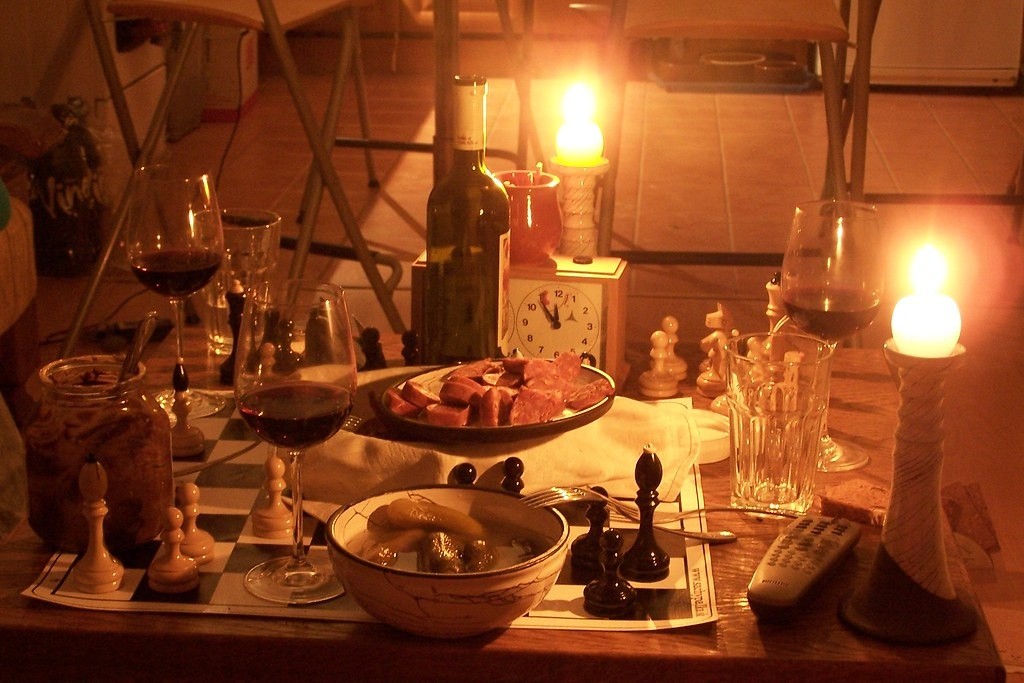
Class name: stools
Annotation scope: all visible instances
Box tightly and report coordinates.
[45,0,1024,362]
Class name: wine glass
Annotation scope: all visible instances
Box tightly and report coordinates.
[780,199,886,471]
[127,164,225,422]
[235,280,357,605]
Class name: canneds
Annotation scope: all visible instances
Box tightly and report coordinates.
[26,354,174,549]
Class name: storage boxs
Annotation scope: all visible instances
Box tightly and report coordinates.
[187,23,260,122]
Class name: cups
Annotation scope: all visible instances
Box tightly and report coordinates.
[499,170,564,263]
[725,331,835,520]
[189,205,281,353]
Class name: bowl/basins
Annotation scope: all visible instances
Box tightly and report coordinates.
[325,486,570,637]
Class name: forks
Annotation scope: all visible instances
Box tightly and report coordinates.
[585,487,801,526]
[522,484,739,541]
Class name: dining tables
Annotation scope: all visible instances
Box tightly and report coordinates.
[0,317,1007,683]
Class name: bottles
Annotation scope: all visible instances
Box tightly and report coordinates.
[425,75,512,365]
[25,353,173,551]
[382,361,614,437]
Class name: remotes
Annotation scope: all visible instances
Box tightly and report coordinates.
[746,514,863,621]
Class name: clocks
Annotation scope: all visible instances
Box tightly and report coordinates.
[507,253,631,395]
